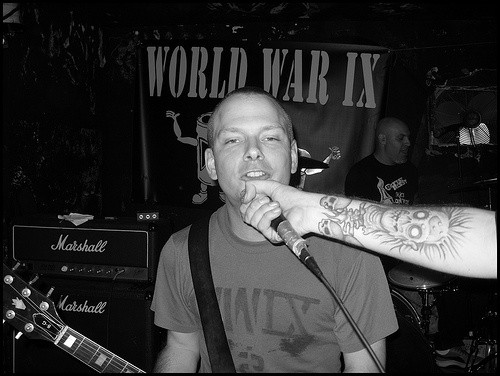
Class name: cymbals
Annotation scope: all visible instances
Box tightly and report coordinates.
[386,263,451,290]
[447,171,499,193]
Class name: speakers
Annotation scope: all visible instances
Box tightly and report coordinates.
[3,218,158,376]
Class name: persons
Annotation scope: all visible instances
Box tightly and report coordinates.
[342,115,439,210]
[238,180,500,279]
[149,88,398,376]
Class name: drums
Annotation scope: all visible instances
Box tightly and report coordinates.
[389,285,428,336]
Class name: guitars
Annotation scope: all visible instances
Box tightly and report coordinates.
[0,265,146,374]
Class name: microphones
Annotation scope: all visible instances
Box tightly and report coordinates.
[242,188,323,278]
